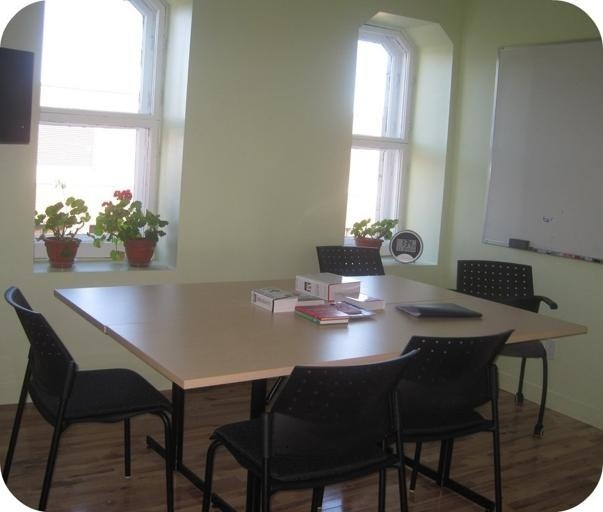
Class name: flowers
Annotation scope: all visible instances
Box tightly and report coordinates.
[88,187,168,261]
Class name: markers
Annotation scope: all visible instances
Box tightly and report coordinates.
[533,247,602,263]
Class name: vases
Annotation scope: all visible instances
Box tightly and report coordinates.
[120,235,158,268]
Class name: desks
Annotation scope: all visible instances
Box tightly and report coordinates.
[49,277,590,510]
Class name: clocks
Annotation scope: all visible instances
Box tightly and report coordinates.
[389,228,423,264]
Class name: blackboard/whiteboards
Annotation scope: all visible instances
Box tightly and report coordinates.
[481,35,603,263]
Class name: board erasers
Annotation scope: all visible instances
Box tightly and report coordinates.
[508,238,529,249]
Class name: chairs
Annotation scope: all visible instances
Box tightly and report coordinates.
[371,328,521,510]
[446,258,560,440]
[202,344,421,511]
[0,283,180,512]
[313,243,387,277]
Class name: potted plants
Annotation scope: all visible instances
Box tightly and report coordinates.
[30,194,92,269]
[346,217,399,253]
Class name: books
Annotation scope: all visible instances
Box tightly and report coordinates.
[248,270,385,325]
